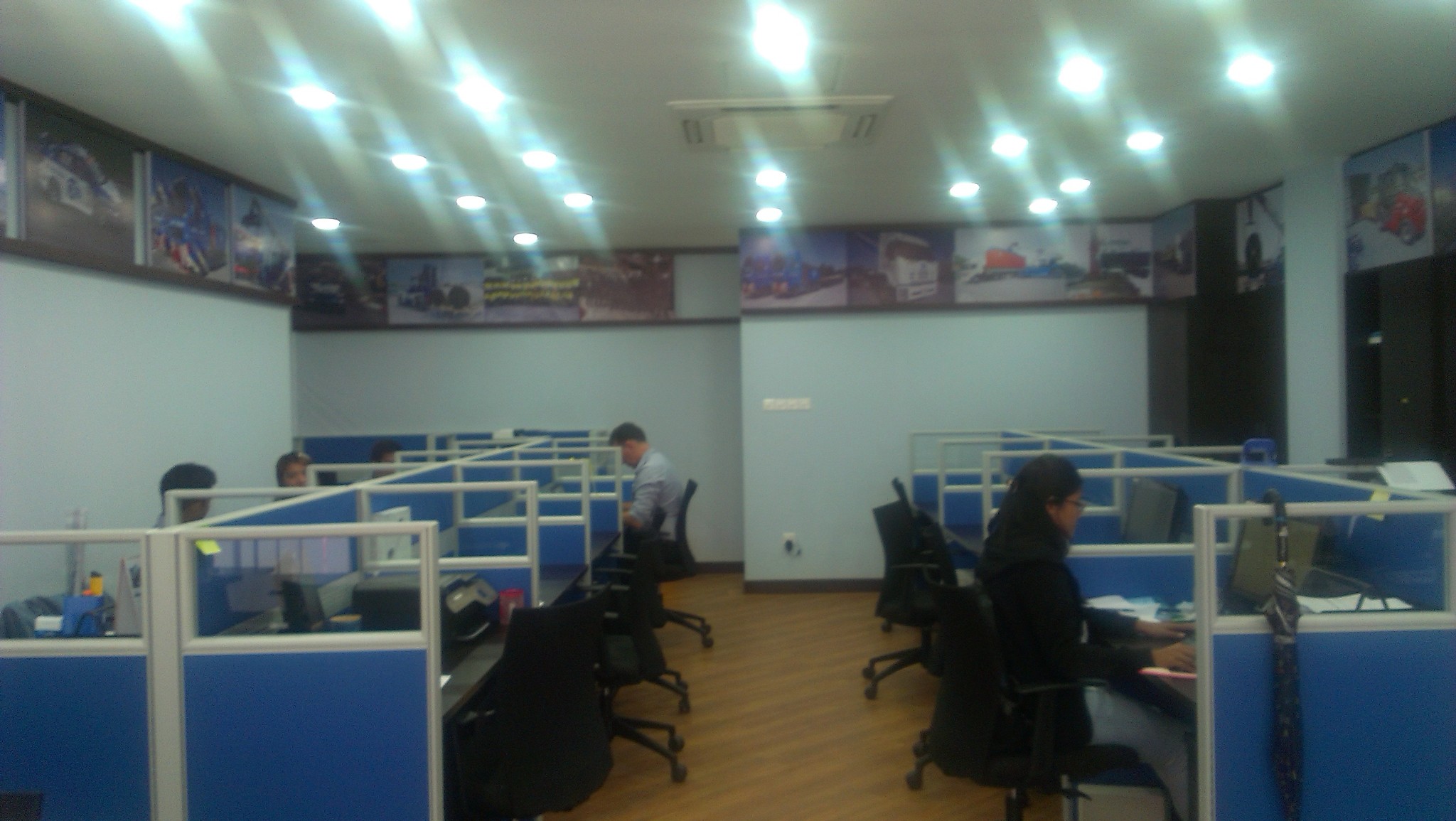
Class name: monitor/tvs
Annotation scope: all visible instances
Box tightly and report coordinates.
[1231,512,1323,610]
[1123,476,1188,543]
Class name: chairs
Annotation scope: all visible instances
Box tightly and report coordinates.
[862,477,1110,821]
[442,479,713,821]
[0,594,65,637]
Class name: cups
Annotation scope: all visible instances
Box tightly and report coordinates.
[500,589,525,625]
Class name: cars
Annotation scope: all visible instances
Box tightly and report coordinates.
[26,139,128,224]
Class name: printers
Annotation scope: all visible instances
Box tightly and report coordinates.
[351,570,499,670]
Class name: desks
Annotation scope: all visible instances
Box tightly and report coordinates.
[541,564,587,607]
[227,571,342,613]
[443,624,509,725]
[591,531,620,563]
[914,500,938,522]
[1106,636,1195,708]
[944,524,983,556]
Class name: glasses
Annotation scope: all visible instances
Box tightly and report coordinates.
[1060,497,1087,517]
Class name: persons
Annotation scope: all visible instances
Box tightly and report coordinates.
[370,439,404,480]
[974,452,1194,818]
[610,420,683,559]
[152,463,218,575]
[275,452,313,488]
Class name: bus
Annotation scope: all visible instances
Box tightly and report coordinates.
[868,231,939,305]
